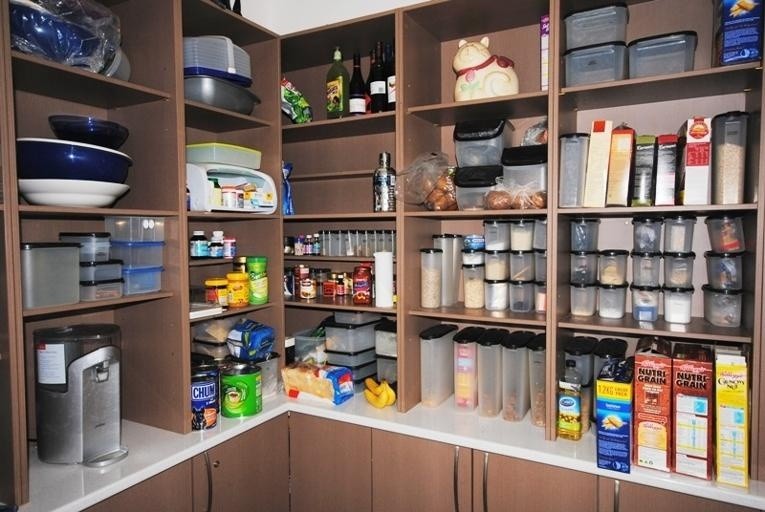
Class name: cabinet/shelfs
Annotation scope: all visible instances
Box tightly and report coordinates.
[0,0,765,511]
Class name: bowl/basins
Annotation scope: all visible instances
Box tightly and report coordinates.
[11,135,135,188]
[47,108,131,148]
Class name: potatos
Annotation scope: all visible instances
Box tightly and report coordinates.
[484,190,547,210]
[418,167,459,211]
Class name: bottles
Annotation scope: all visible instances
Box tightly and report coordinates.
[190,228,236,259]
[557,357,586,444]
[291,228,322,260]
[420,219,549,315]
[283,263,399,308]
[324,35,401,121]
[372,147,397,220]
[571,215,745,329]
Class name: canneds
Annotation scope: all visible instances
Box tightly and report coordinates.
[233,256,246,272]
[205,278,229,312]
[226,273,249,307]
[353,266,372,305]
[190,353,262,432]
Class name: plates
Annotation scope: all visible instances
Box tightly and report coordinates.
[17,174,133,211]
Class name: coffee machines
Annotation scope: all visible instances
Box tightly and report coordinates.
[31,320,138,475]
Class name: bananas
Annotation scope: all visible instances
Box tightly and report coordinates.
[364,377,397,409]
